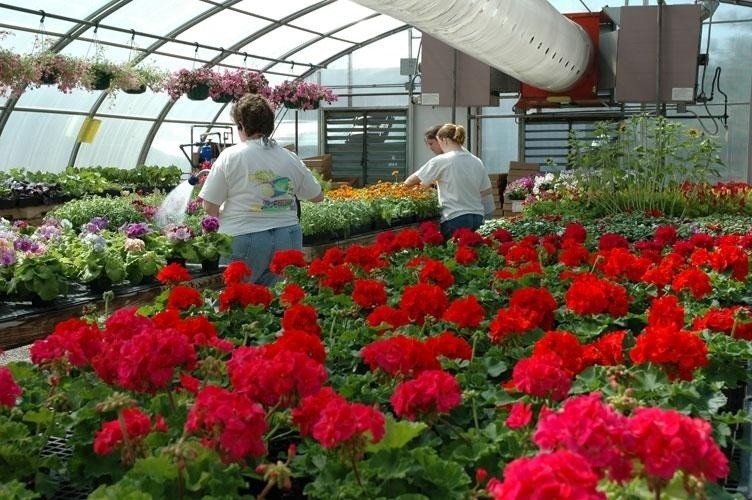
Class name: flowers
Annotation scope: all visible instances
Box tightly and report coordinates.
[320,168,441,225]
[0,212,237,300]
[269,80,339,109]
[503,170,554,200]
[1,40,116,94]
[110,57,165,95]
[169,68,272,103]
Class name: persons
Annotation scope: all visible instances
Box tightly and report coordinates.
[423,125,496,221]
[405,123,484,241]
[197,92,322,288]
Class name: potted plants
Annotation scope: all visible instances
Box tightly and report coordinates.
[300,200,327,245]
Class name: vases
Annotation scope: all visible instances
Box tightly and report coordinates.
[510,201,525,212]
[122,82,148,95]
